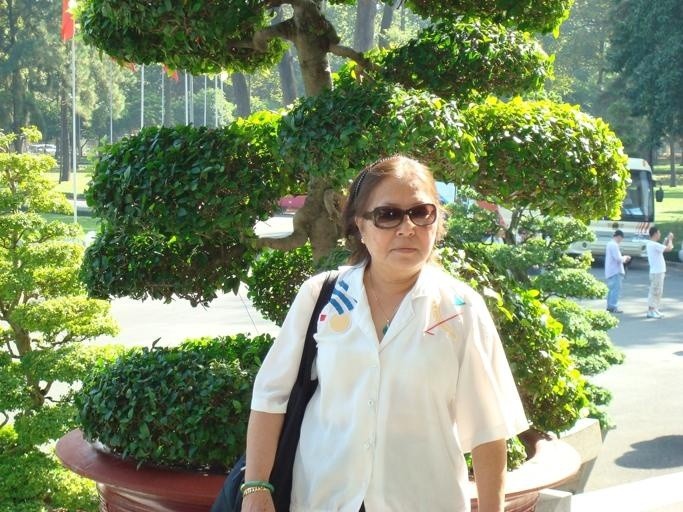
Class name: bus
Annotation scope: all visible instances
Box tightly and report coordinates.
[432,156,663,261]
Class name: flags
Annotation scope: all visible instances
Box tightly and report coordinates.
[164,66,179,82]
[112,57,137,72]
[60,0,74,44]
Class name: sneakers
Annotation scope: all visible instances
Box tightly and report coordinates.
[606,307,664,319]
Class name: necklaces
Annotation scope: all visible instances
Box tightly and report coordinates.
[368,269,420,336]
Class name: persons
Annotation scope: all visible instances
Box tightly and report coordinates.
[603,230,631,313]
[514,226,527,243]
[644,227,673,319]
[528,260,543,275]
[482,224,506,246]
[233,155,529,512]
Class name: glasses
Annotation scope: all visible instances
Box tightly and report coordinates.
[361,204,436,229]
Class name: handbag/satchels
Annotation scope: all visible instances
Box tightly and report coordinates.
[208,441,296,512]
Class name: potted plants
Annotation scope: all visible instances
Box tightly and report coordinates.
[54,0,629,512]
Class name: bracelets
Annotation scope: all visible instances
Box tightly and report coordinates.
[241,487,270,496]
[238,481,274,493]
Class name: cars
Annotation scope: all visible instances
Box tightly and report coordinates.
[29,143,59,156]
